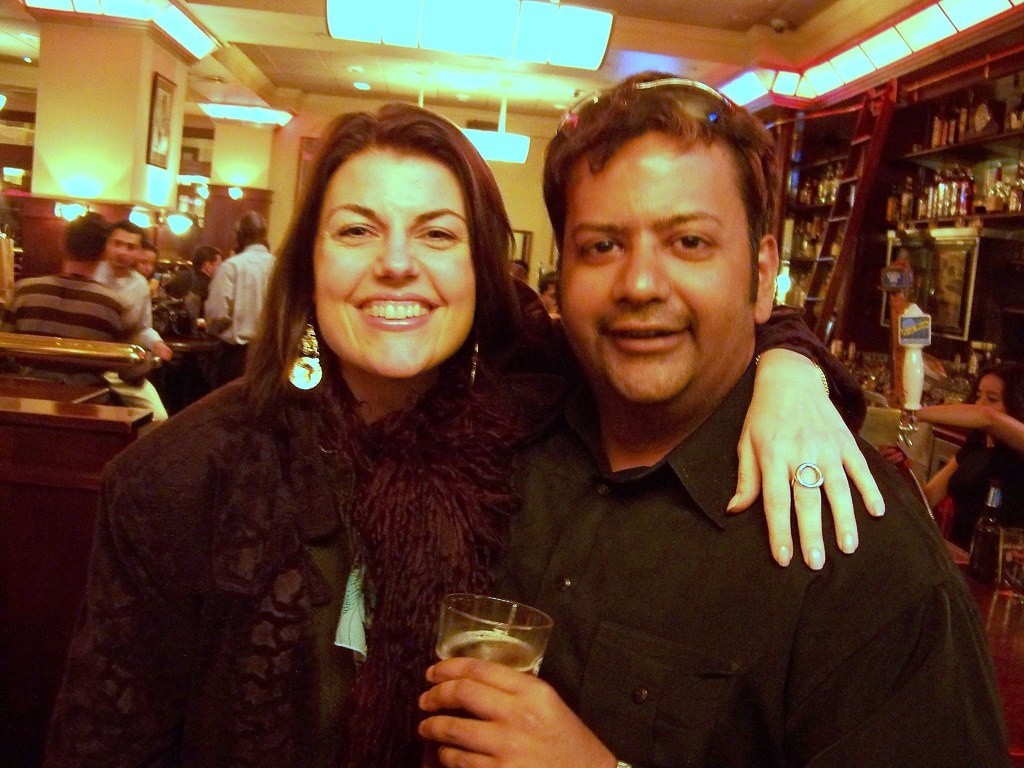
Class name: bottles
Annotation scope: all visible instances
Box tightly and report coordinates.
[967,483,1000,589]
[918,169,975,218]
[801,164,843,205]
[986,165,1024,212]
[932,106,956,147]
[886,178,913,222]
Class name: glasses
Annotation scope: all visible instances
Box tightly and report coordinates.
[559,76,733,141]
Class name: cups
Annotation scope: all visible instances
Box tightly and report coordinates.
[197,319,205,336]
[422,593,554,768]
[1001,528,1024,597]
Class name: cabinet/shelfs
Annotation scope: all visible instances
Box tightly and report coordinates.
[889,74,1024,356]
[772,96,884,313]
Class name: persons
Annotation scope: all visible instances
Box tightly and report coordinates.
[418,71,1014,768]
[0,210,280,422]
[878,362,1024,553]
[44,103,886,768]
[510,259,561,322]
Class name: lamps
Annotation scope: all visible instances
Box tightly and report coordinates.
[419,71,532,165]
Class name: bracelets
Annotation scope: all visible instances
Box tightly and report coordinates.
[617,761,632,768]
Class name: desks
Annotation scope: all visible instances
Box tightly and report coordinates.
[157,335,230,388]
[0,335,144,389]
[954,563,1024,768]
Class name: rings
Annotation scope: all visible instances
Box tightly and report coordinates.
[790,459,826,493]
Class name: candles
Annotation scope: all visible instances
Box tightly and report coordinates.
[782,219,795,263]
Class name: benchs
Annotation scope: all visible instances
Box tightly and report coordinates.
[0,396,156,495]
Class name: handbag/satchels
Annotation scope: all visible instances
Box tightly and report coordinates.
[153,299,199,340]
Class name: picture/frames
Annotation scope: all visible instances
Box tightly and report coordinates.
[510,228,534,267]
[148,72,179,171]
[880,229,980,341]
